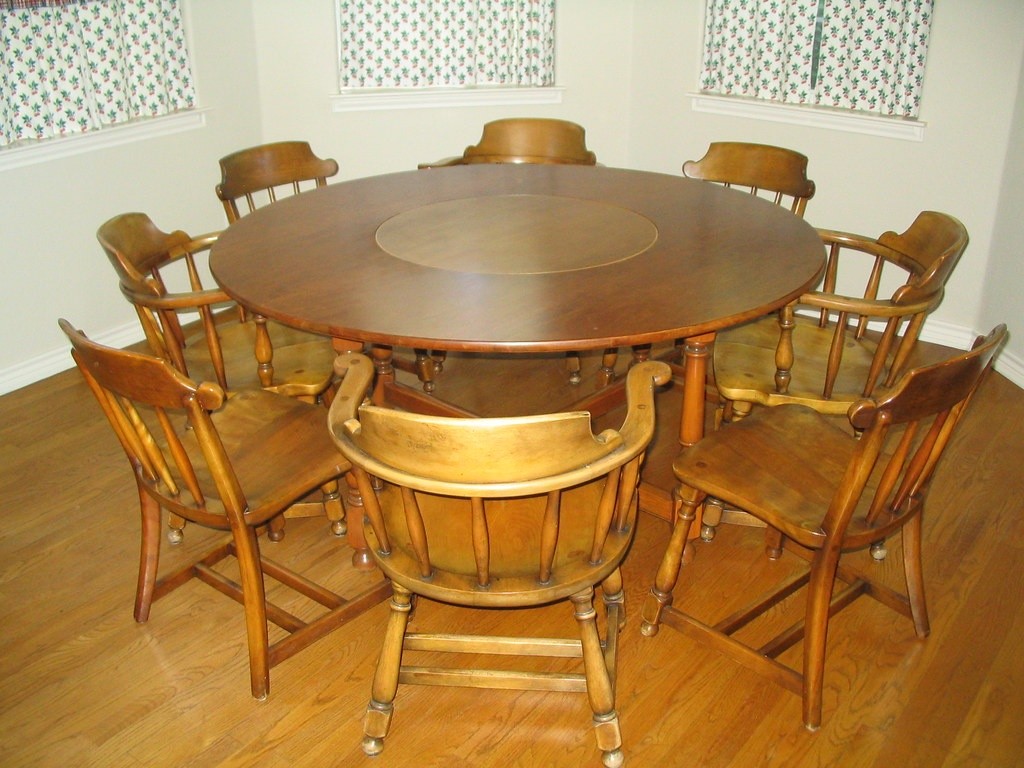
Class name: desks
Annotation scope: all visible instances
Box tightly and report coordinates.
[208,161,828,571]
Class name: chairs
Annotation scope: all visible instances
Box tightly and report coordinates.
[329,353,674,768]
[638,322,1008,731]
[216,141,338,227]
[95,210,348,545]
[418,118,596,168]
[700,210,970,542]
[682,142,816,218]
[59,318,418,701]
